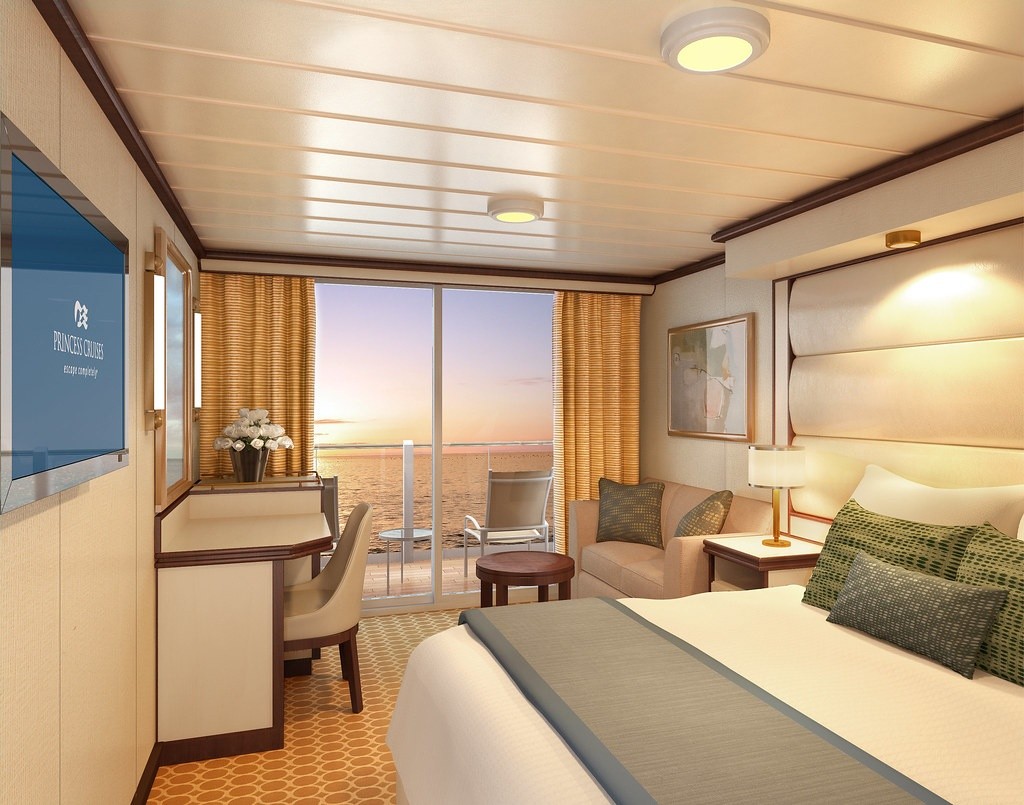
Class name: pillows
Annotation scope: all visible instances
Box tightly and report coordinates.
[675,490,733,538]
[596,478,665,550]
[801,463,1024,687]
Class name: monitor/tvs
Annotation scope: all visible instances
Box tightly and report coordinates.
[0,112,130,517]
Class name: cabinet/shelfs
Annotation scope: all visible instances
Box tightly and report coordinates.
[188,471,340,677]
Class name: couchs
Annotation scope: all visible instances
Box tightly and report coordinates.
[568,476,772,600]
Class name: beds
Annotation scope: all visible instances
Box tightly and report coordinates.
[386,582,1024,805]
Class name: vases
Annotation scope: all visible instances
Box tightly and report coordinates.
[229,445,270,483]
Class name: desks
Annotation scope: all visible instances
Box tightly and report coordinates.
[379,529,432,595]
[154,489,333,767]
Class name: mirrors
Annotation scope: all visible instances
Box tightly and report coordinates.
[155,227,193,513]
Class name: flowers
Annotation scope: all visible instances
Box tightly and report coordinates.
[215,408,295,453]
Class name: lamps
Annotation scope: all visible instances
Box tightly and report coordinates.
[488,200,544,223]
[661,6,771,73]
[144,251,165,431]
[885,230,921,249]
[192,297,202,422]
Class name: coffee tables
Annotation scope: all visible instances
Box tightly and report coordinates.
[476,551,576,607]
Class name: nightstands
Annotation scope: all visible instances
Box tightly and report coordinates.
[703,533,822,593]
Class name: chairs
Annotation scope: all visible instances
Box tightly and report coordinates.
[464,466,555,579]
[283,501,372,714]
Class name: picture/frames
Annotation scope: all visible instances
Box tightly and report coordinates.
[667,311,755,443]
